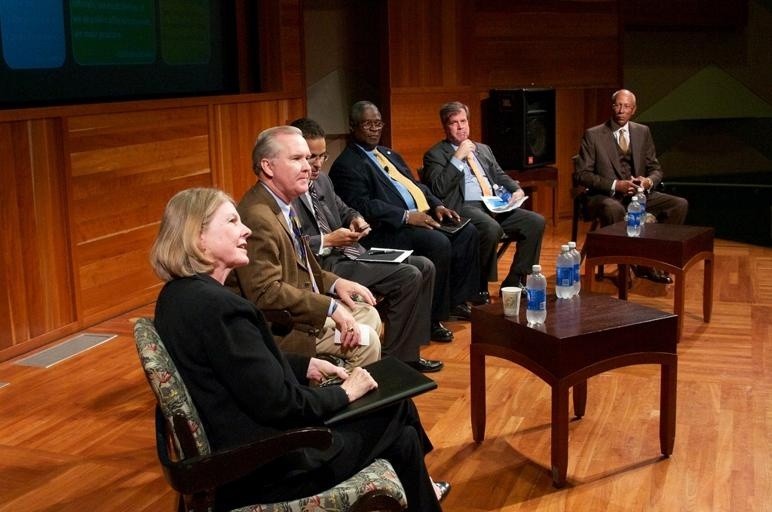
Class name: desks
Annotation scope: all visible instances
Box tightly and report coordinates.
[585,218,714,344]
[469,292,678,486]
[505,166,559,227]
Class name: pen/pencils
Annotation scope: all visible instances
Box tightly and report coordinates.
[368,250,399,255]
[320,367,355,386]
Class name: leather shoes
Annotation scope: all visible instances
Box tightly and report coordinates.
[469,290,491,306]
[434,479,452,502]
[448,302,473,319]
[428,320,454,343]
[409,356,445,372]
[499,278,529,297]
[637,266,673,284]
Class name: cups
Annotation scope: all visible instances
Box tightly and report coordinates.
[501,286,522,316]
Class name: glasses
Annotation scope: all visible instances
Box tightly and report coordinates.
[308,152,329,162]
[352,119,384,131]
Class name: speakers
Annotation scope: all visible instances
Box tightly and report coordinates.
[480,88,556,170]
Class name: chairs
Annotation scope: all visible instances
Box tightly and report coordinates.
[129,316,409,512]
[570,154,606,280]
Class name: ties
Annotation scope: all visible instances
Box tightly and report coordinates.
[618,129,628,153]
[288,207,321,297]
[308,180,362,257]
[464,152,491,196]
[371,147,431,213]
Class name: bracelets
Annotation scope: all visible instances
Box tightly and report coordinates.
[330,297,339,315]
[405,207,411,224]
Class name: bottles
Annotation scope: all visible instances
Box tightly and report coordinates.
[634,188,647,226]
[556,245,574,299]
[491,184,511,202]
[627,197,641,237]
[567,242,581,296]
[526,265,547,324]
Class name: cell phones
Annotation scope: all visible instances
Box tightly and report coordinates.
[355,224,370,232]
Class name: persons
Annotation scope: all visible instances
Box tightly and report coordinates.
[577,87,687,288]
[150,187,453,511]
[425,100,546,308]
[289,116,445,370]
[234,123,383,375]
[328,98,476,340]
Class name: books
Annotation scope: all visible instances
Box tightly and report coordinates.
[321,353,438,426]
[434,214,471,235]
[353,248,412,263]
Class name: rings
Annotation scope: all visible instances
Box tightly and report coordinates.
[424,219,428,223]
[349,324,355,332]
